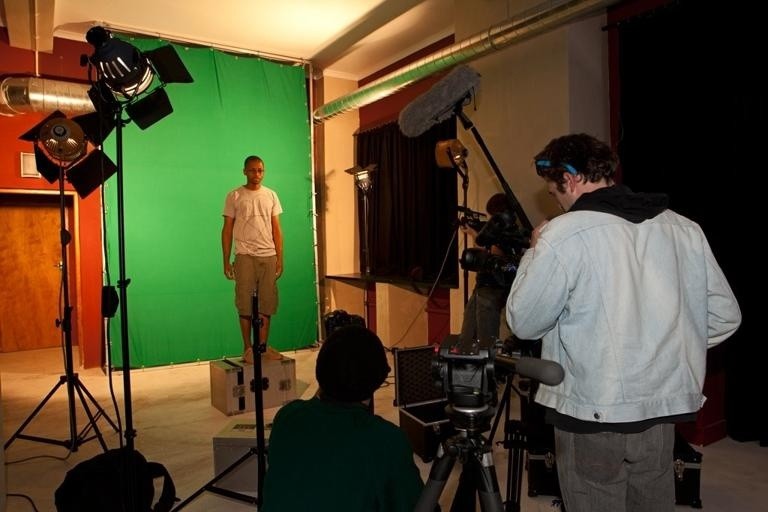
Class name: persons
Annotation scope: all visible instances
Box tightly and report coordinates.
[456,191,524,354]
[220,155,291,363]
[505,132,744,512]
[258,322,445,511]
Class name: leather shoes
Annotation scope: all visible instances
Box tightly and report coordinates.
[243,347,254,364]
[261,345,283,360]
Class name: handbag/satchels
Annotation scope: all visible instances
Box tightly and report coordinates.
[54,445,176,511]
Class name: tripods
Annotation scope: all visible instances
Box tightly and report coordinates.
[3,175,120,457]
[171,289,269,512]
[412,432,504,512]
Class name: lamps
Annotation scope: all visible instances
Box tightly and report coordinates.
[6,25,193,455]
[436,140,468,306]
[345,164,379,330]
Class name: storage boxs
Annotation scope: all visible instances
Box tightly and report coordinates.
[210,349,296,498]
[524,438,703,509]
[393,346,455,464]
